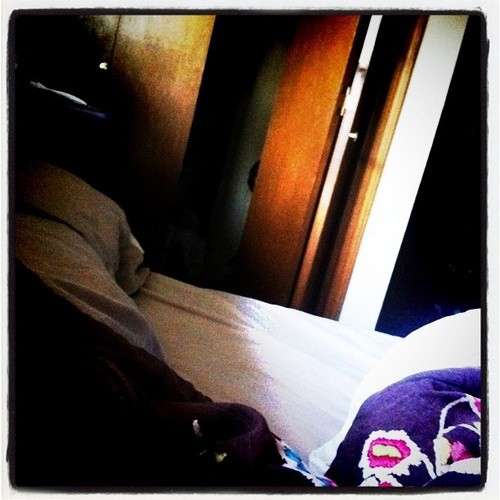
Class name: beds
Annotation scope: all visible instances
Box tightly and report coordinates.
[13,266,481,486]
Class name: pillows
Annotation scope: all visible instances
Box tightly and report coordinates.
[10,155,151,296]
[10,212,166,360]
